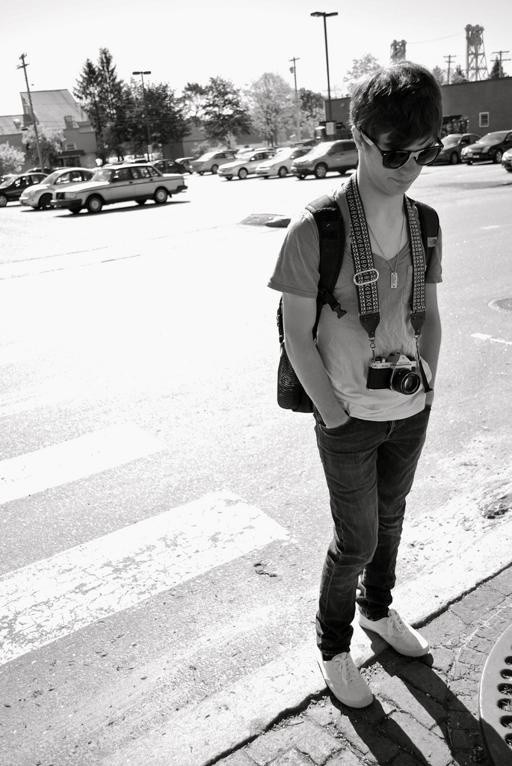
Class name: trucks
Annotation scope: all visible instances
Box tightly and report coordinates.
[290,123,353,150]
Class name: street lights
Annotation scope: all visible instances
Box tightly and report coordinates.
[309,9,339,120]
[131,69,153,161]
[16,53,47,175]
[289,56,299,105]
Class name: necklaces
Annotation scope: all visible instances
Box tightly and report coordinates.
[365,203,406,289]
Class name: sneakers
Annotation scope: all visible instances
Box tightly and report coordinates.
[316,651,373,708]
[359,609,429,657]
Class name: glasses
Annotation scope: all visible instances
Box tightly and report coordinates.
[361,132,444,168]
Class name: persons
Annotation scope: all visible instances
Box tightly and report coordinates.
[267,61,443,710]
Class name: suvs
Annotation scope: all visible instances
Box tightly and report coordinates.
[292,138,359,180]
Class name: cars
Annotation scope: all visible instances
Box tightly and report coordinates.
[19,165,96,210]
[191,150,240,176]
[426,133,481,166]
[503,147,512,172]
[176,157,196,174]
[0,172,49,208]
[240,147,289,154]
[256,147,312,179]
[461,129,512,165]
[219,150,277,181]
[146,159,187,175]
[51,163,188,215]
[1,166,64,182]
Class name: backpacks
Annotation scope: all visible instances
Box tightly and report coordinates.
[276,198,439,413]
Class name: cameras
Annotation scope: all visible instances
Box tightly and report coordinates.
[367,351,422,396]
